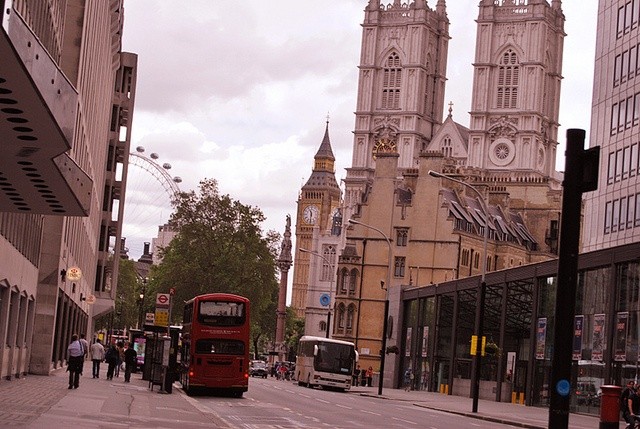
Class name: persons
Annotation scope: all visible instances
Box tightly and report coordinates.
[403,369,411,392]
[78,333,89,375]
[91,338,104,379]
[123,343,137,382]
[269,362,291,381]
[105,344,120,380]
[66,334,84,389]
[351,365,361,387]
[622,380,636,423]
[366,366,375,387]
[627,384,640,428]
[114,342,125,377]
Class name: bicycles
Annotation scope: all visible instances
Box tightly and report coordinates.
[624,413,640,429]
[277,371,287,380]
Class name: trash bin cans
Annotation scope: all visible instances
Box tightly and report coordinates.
[599,385,622,428]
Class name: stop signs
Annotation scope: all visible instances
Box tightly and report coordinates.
[156,293,170,305]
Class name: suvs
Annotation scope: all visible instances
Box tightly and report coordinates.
[251,361,268,379]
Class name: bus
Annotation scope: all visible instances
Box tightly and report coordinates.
[578,361,639,392]
[179,293,250,397]
[108,335,129,352]
[294,336,359,393]
[169,325,183,363]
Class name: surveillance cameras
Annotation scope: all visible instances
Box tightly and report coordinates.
[380,280,387,291]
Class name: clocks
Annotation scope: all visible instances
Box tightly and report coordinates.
[301,202,321,227]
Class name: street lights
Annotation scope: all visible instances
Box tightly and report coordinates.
[138,277,147,330]
[298,248,334,338]
[428,169,489,412]
[347,219,394,396]
